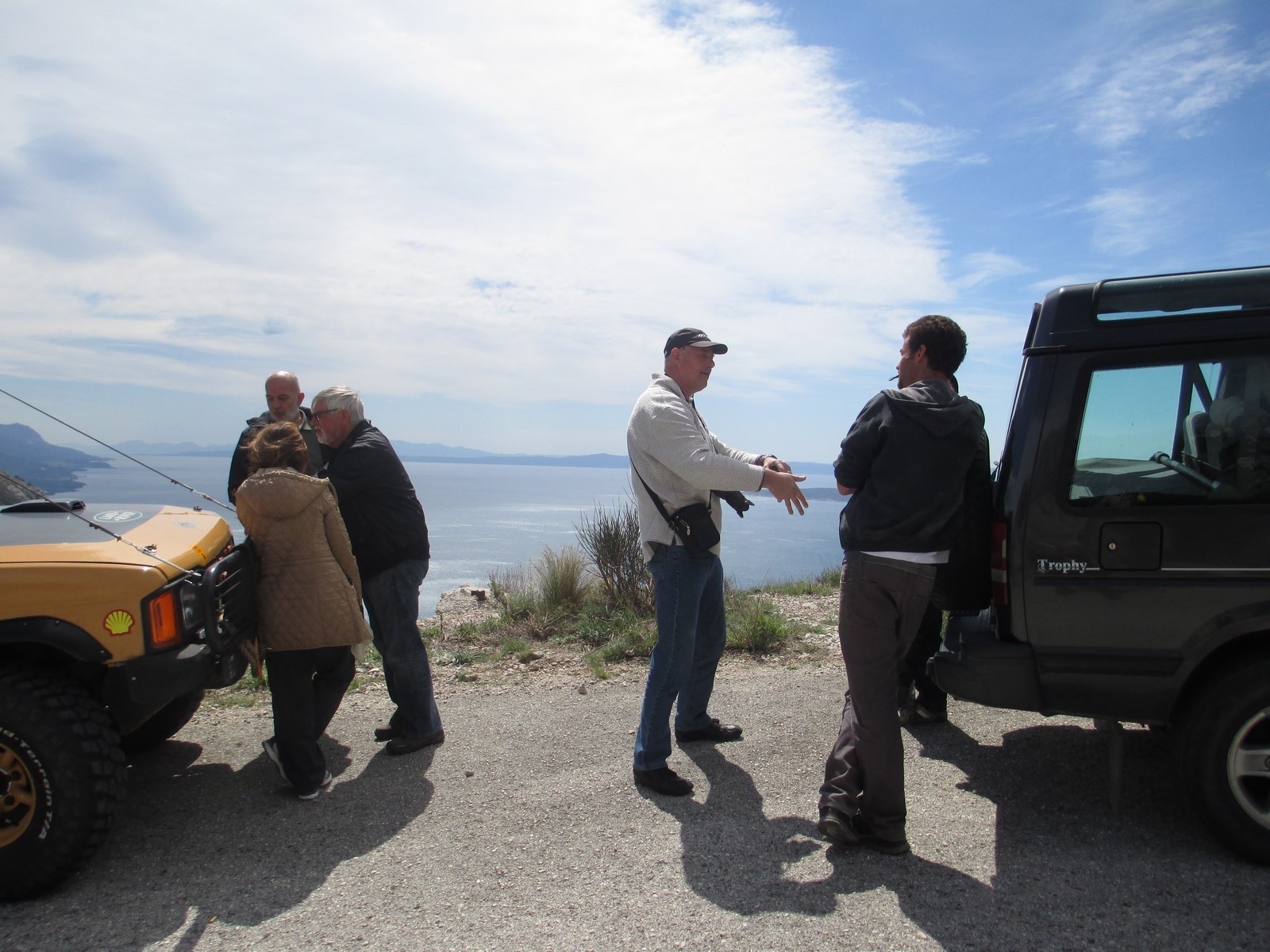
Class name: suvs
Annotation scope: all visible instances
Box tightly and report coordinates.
[0,492,259,906]
[926,264,1270,867]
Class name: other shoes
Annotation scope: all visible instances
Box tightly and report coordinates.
[261,738,287,782]
[386,728,443,756]
[297,784,319,799]
[322,769,331,786]
[896,702,947,726]
[852,810,911,854]
[817,808,859,843]
[374,723,397,738]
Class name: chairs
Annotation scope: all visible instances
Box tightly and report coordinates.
[1205,397,1249,484]
[1182,411,1210,460]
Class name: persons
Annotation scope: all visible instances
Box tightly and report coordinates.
[626,328,810,797]
[236,421,374,799]
[309,386,444,756]
[815,315,985,857]
[227,371,332,507]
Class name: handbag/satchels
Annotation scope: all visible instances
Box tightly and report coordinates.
[667,502,720,554]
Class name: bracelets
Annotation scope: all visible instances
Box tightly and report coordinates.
[754,454,764,465]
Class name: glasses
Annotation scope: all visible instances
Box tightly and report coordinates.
[311,407,339,421]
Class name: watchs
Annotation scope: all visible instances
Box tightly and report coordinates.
[759,454,777,466]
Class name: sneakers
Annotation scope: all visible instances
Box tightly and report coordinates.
[633,766,694,796]
[675,718,742,744]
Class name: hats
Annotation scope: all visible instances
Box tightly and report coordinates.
[664,328,728,358]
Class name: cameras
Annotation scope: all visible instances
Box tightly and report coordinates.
[712,490,755,518]
